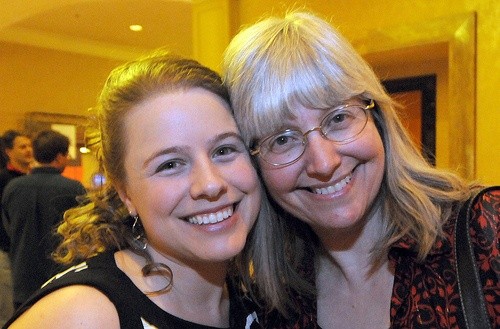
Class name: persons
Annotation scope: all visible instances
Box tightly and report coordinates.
[0,129,90,311]
[2,45,267,329]
[0,131,33,329]
[221,11,500,329]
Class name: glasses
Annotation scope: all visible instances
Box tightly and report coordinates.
[248,100,374,166]
